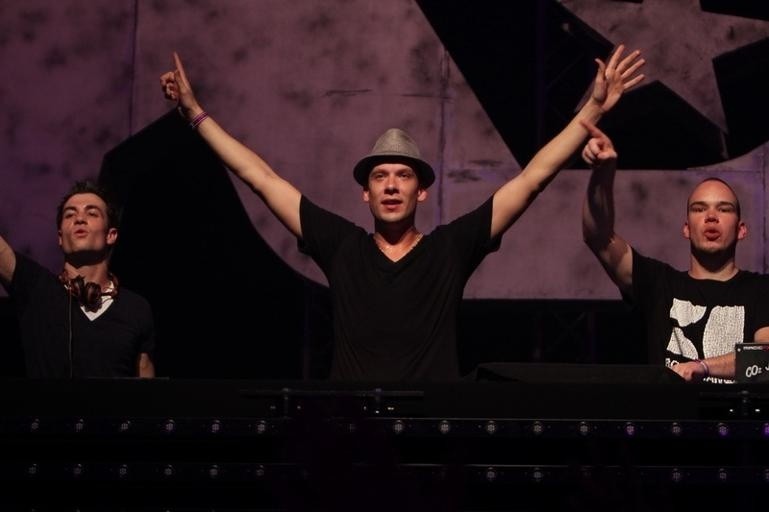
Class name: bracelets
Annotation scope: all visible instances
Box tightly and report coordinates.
[697,359,710,379]
[189,110,209,129]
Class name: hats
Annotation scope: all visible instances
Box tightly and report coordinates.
[352,127,435,189]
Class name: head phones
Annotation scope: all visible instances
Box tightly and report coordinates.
[62,261,119,310]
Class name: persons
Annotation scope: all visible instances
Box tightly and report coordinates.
[579,119,768,382]
[0,179,157,379]
[160,44,645,380]
